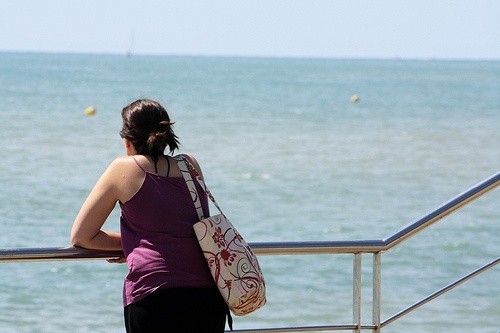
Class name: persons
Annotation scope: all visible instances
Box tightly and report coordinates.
[71,98,236,333]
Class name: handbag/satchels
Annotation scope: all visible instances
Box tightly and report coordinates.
[174,153,266,317]
